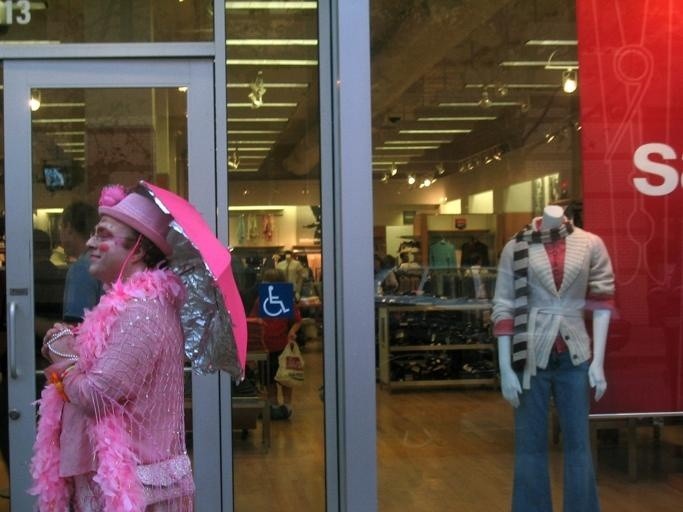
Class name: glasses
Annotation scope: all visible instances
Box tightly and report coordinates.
[90,231,137,241]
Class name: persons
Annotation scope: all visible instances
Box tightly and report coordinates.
[26,194,196,512]
[32,228,63,401]
[488,205,616,512]
[277,253,306,300]
[246,268,303,421]
[377,255,400,296]
[35,200,105,339]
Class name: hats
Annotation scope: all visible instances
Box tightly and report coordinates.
[98,192,175,257]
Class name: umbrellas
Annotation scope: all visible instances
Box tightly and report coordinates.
[124,180,248,385]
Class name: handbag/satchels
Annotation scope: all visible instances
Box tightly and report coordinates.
[274,341,305,388]
[135,455,195,506]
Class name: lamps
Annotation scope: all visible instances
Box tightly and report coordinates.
[228,150,240,169]
[246,78,266,109]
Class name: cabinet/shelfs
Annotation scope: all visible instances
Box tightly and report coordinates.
[377,305,500,394]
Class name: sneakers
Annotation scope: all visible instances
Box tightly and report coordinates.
[258,404,292,420]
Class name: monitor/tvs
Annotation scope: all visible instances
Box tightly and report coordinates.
[38,157,77,191]
